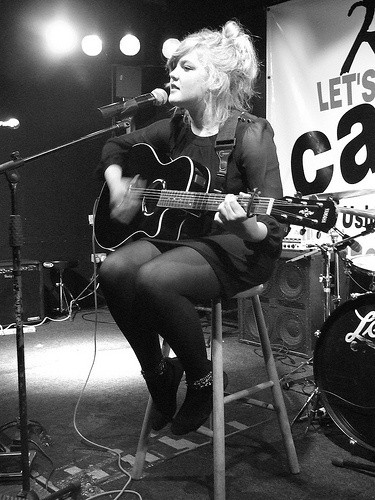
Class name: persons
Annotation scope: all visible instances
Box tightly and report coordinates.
[97,19,289,435]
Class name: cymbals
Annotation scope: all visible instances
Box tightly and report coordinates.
[299,188,375,203]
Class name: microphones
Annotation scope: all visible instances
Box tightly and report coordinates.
[97,88,167,120]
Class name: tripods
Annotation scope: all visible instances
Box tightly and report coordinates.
[0,117,81,500]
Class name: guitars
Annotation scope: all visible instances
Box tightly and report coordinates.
[91,142,337,251]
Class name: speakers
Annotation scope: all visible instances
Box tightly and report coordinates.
[0,260,44,326]
[237,249,348,359]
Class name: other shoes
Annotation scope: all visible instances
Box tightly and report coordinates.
[170,360,228,435]
[141,356,184,431]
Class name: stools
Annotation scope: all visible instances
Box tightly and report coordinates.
[130,285,300,500]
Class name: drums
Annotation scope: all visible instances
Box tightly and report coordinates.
[343,254,375,298]
[313,291,375,453]
